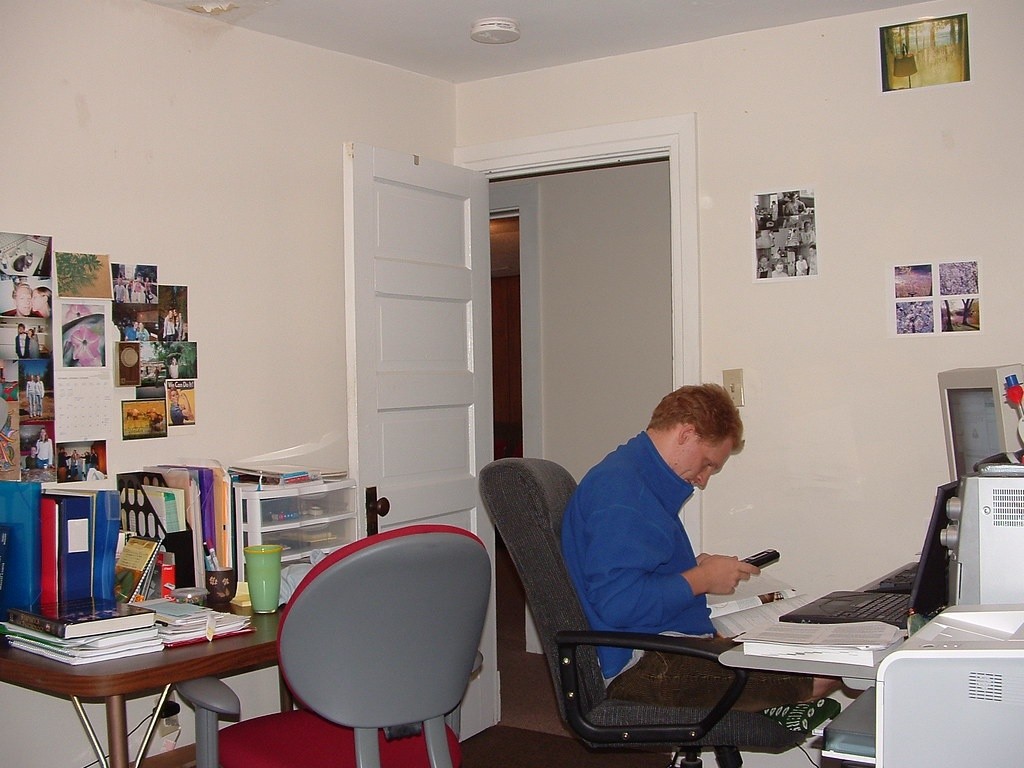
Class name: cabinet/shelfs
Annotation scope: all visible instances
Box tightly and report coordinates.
[231,477,358,595]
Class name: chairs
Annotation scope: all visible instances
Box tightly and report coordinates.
[479,456,841,768]
[174,523,491,768]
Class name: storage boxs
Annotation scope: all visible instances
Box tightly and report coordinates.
[146,553,174,600]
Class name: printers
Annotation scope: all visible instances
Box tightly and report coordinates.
[875,604,1023,768]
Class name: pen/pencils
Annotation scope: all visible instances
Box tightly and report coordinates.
[202,538,221,571]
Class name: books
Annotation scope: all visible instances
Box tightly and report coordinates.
[114,535,163,604]
[130,597,255,651]
[229,461,348,487]
[705,572,821,638]
[0,597,166,666]
[38,497,59,619]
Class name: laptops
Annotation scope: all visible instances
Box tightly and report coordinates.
[779,480,959,630]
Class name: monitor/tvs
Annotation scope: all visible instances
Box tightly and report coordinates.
[937,363,1024,486]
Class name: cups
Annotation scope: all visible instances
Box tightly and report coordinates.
[205,566,237,605]
[244,544,282,613]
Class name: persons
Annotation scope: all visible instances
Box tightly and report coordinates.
[168,388,193,425]
[26,374,36,418]
[559,381,844,711]
[125,322,149,341]
[24,428,54,469]
[154,366,160,387]
[15,322,40,359]
[11,283,39,317]
[31,287,52,317]
[169,357,179,379]
[36,375,44,417]
[163,310,186,340]
[113,274,152,303]
[756,192,817,279]
[57,447,99,483]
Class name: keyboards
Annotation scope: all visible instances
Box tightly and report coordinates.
[863,562,920,594]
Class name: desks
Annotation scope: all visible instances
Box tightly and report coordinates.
[0,604,294,768]
[22,468,55,482]
[718,561,918,768]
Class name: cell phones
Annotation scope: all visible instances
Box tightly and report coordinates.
[739,550,780,567]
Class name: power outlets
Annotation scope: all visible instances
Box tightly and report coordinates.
[153,693,180,737]
